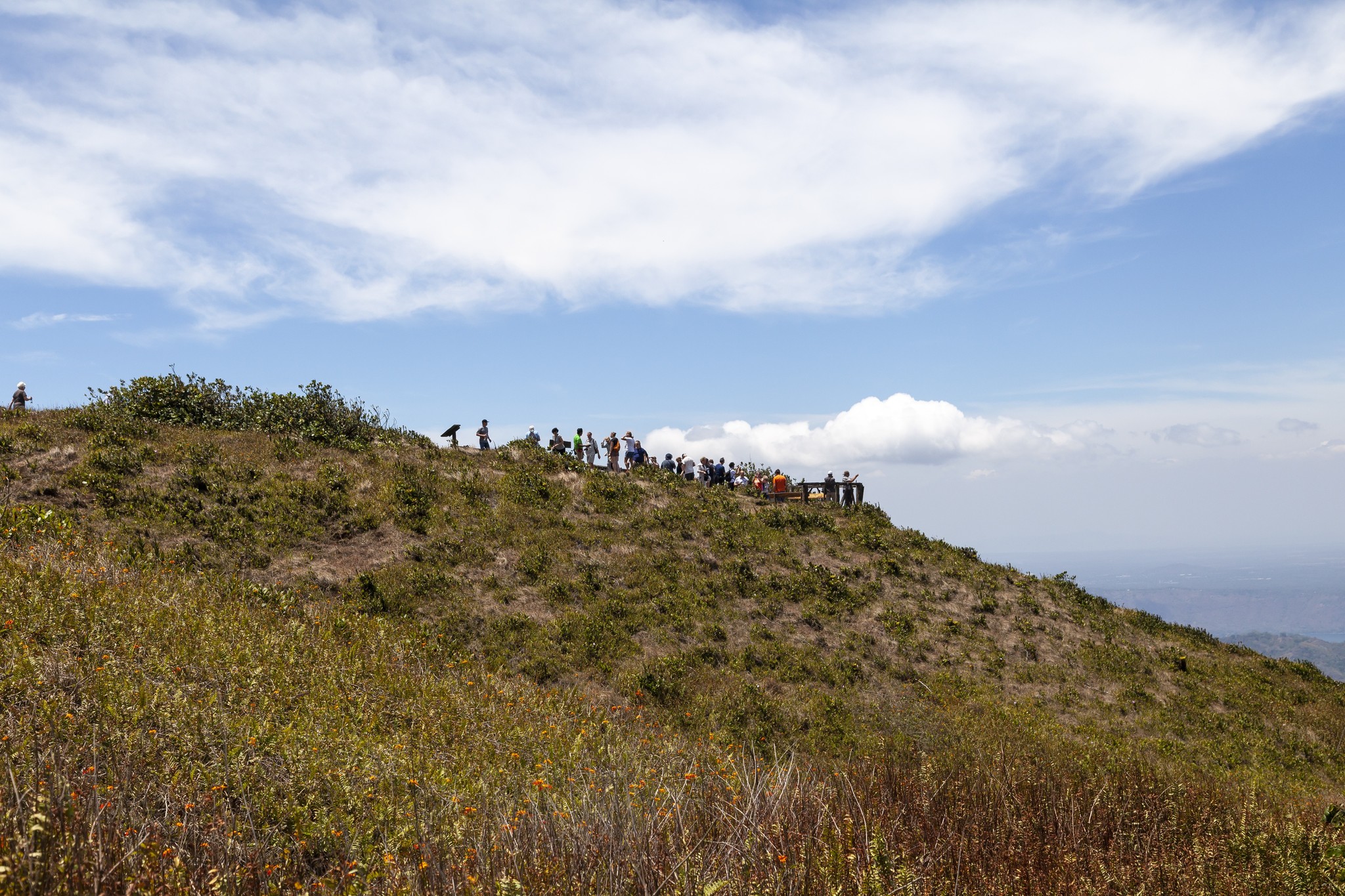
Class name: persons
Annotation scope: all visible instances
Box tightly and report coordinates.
[715,457,726,485]
[682,453,695,481]
[525,426,541,448]
[728,462,739,491]
[8,382,32,411]
[675,457,685,480]
[476,419,491,450]
[632,441,649,468]
[646,456,659,468]
[601,437,614,471]
[697,457,715,489]
[584,432,601,469]
[661,453,676,472]
[551,427,566,456]
[824,471,837,505]
[734,470,749,487]
[842,471,859,507]
[762,475,770,502]
[621,431,637,472]
[753,471,764,492]
[574,428,590,460]
[773,469,788,504]
[609,432,620,474]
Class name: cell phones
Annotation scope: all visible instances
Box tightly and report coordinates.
[588,445,591,447]
[738,467,741,468]
[649,457,651,459]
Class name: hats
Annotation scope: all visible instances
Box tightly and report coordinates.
[482,419,489,423]
[682,453,686,460]
[828,471,832,474]
[649,456,656,459]
[843,471,849,475]
[665,453,672,459]
[609,456,613,463]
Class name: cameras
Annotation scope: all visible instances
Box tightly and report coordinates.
[584,447,588,451]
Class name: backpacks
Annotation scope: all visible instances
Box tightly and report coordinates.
[586,439,597,454]
[529,432,537,445]
[725,469,734,482]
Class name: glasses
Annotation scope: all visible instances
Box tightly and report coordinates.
[677,460,680,462]
[636,444,638,445]
[757,474,760,475]
[587,435,590,436]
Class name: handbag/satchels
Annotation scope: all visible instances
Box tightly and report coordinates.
[708,466,714,477]
[613,440,621,452]
[704,476,708,481]
[821,487,827,495]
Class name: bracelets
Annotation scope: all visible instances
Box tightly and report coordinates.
[652,460,653,462]
[609,452,611,453]
[702,471,703,473]
[598,453,600,455]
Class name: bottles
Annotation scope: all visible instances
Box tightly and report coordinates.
[29,396,32,404]
[707,467,709,474]
[484,433,487,440]
[708,475,710,482]
